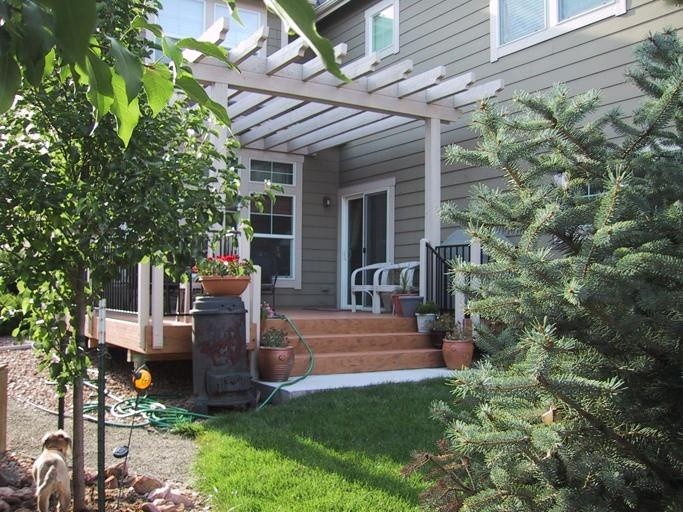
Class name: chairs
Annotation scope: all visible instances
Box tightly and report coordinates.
[350,260,424,313]
[250,247,280,310]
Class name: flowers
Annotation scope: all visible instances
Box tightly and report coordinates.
[191,255,257,277]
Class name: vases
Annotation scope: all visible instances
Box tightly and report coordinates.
[198,275,250,296]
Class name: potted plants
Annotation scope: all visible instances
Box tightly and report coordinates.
[414,301,441,333]
[391,264,417,315]
[442,321,475,370]
[259,326,296,381]
[398,283,425,317]
[428,313,454,349]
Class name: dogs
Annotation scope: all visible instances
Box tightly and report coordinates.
[31,429,73,512]
